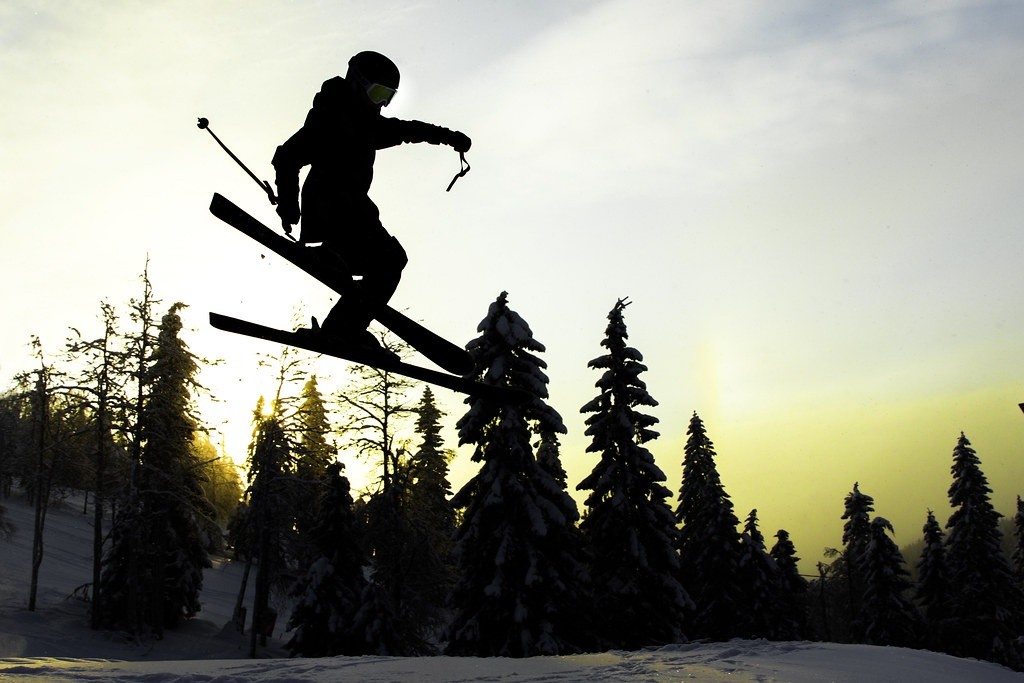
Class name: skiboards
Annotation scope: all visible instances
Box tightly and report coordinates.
[209,312,563,427]
[208,191,474,375]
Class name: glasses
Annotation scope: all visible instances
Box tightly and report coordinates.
[365,83,395,106]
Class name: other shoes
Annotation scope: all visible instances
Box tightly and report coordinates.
[317,327,380,349]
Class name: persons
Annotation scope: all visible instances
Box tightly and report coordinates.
[273,50,472,353]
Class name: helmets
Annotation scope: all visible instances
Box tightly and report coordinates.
[347,51,400,89]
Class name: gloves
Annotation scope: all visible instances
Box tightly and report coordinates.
[278,195,299,225]
[453,131,471,152]
